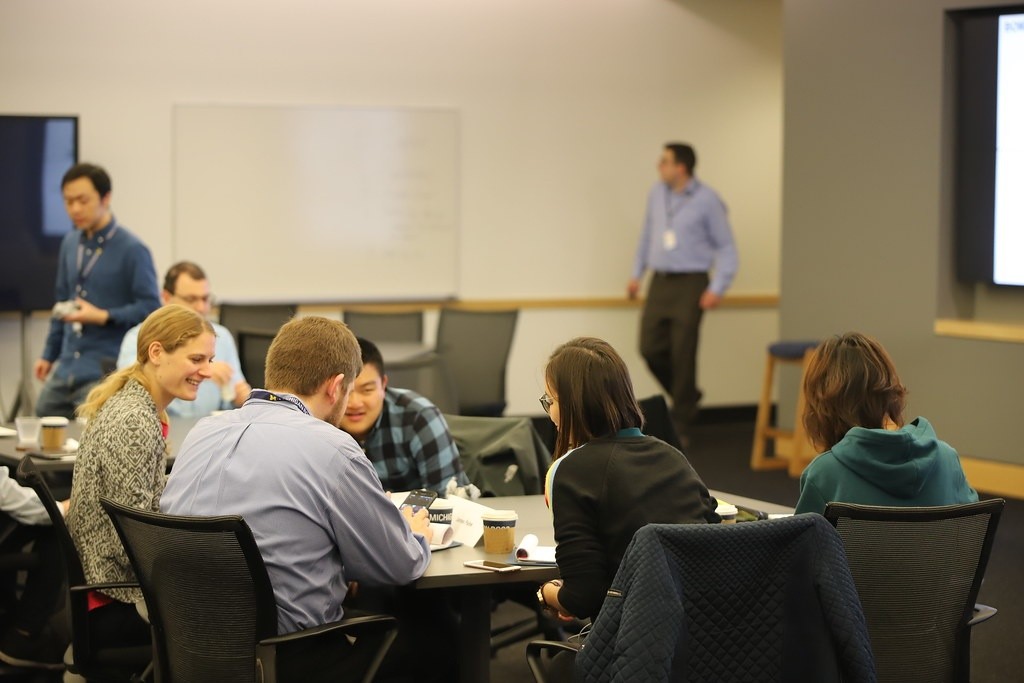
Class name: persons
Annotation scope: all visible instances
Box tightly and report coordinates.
[36,164,160,421]
[535,336,723,683]
[70,261,472,683]
[0,466,72,669]
[794,330,980,683]
[626,143,736,446]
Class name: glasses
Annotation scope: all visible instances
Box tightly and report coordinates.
[539,393,558,413]
[174,292,209,303]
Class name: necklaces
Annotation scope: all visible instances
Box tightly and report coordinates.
[883,423,896,425]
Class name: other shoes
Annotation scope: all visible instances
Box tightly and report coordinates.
[676,433,692,451]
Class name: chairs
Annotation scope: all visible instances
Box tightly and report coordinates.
[0,301,1005,683]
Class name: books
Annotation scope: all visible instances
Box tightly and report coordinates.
[27,449,76,459]
[515,534,556,563]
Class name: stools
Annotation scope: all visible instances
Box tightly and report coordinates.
[750,340,824,471]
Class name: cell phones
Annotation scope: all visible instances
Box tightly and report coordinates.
[398,489,437,518]
[463,560,522,573]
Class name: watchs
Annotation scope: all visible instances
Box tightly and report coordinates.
[537,581,561,610]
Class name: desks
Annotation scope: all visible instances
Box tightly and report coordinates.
[416,489,797,683]
[0,418,200,503]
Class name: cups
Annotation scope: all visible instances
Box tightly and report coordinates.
[15,416,43,450]
[40,417,69,450]
[481,510,518,554]
[427,508,453,526]
[720,514,736,525]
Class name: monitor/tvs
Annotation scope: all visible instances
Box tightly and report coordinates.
[0,115,80,312]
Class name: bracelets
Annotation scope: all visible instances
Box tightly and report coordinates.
[99,317,111,328]
